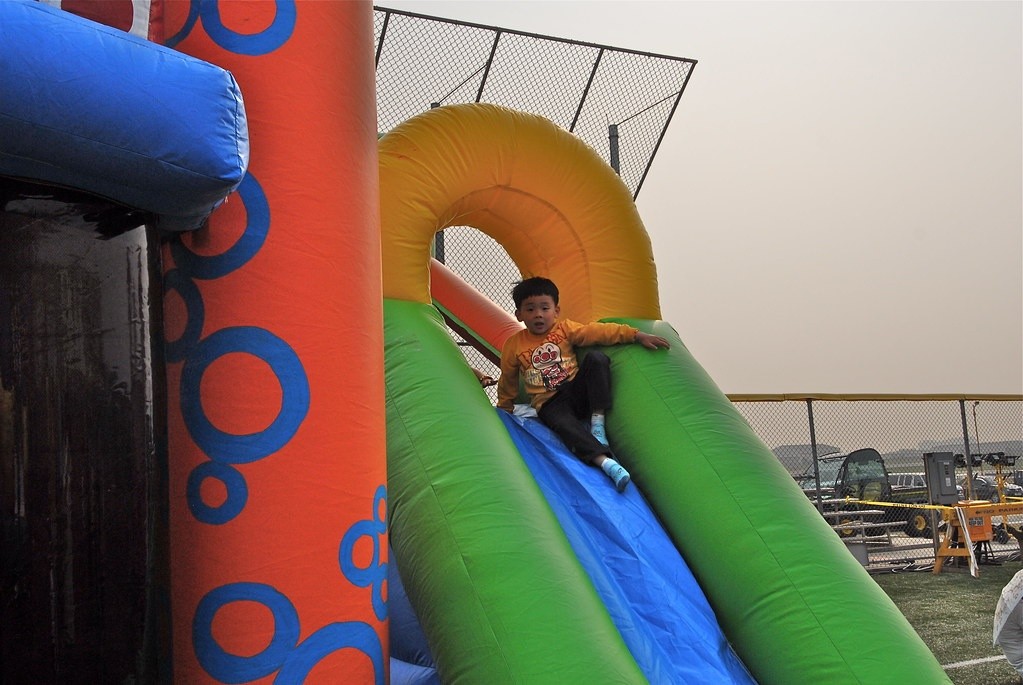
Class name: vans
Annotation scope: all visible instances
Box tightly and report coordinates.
[885,472,966,500]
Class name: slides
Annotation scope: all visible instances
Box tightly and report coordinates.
[378,301,955,685]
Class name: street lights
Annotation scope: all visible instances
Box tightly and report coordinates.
[973,402,983,475]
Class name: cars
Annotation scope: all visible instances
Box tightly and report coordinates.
[957,475,1023,504]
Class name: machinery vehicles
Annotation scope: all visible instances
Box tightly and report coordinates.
[796,447,929,540]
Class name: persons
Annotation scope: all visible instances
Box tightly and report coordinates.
[496,276,671,493]
[472,368,492,386]
